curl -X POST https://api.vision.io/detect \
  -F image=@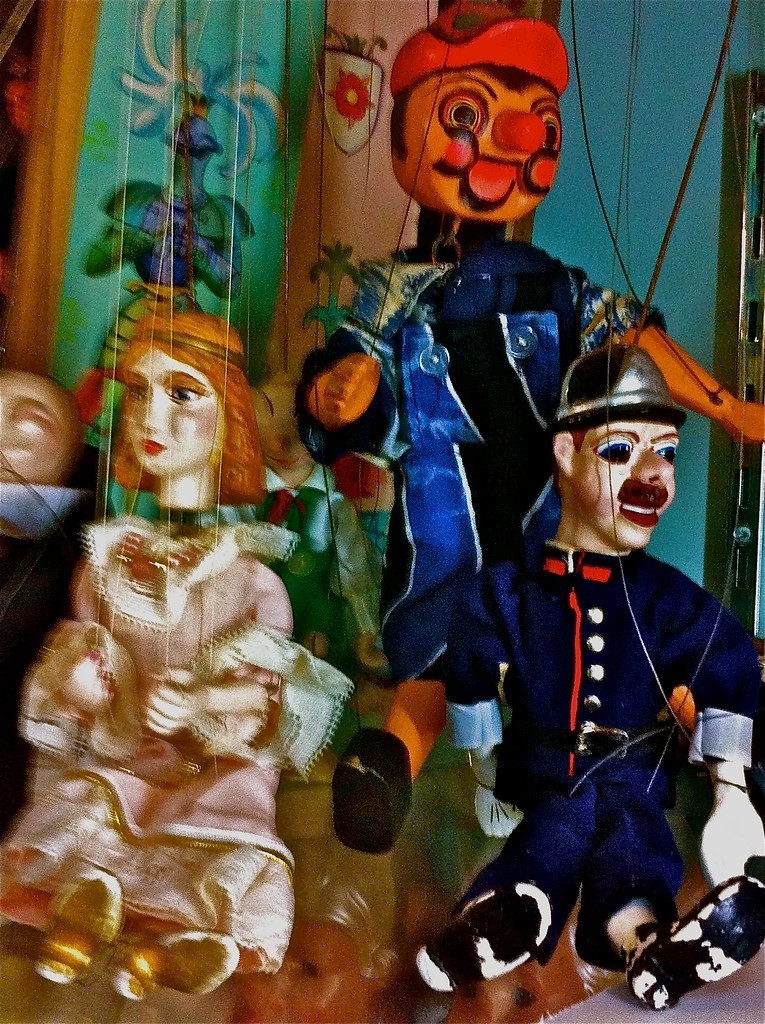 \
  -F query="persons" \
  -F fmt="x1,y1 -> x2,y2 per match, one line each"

417,339 -> 765,1011
0,312 -> 353,1001
293,2 -> 765,859
